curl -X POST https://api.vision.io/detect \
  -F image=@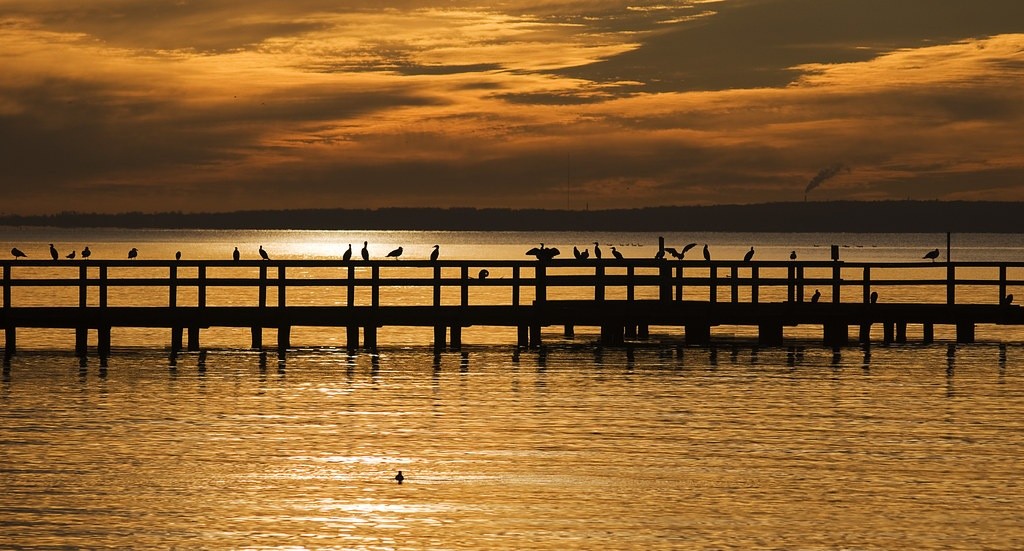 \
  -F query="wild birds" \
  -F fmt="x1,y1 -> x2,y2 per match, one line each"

430,245 -> 439,260
65,247 -> 91,259
573,242 -> 624,260
655,243 -> 711,261
233,247 -> 240,260
128,248 -> 138,259
790,251 -> 797,261
342,244 -> 352,260
871,291 -> 878,304
526,243 -> 560,260
176,251 -> 181,260
744,247 -> 754,261
49,244 -> 58,260
395,471 -> 404,480
259,245 -> 270,260
811,289 -> 821,303
11,248 -> 28,260
922,249 -> 939,262
385,247 -> 403,260
361,241 -> 369,260
